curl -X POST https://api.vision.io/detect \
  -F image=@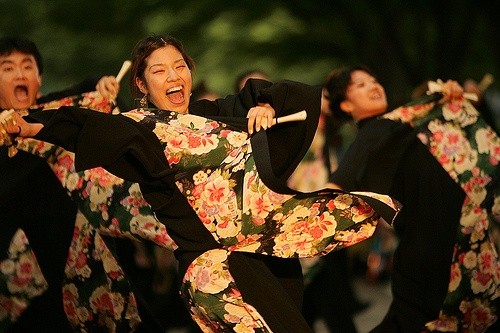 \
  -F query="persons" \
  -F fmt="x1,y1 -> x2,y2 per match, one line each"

0,33 -> 315,333
320,66 -> 500,333
235,64 -> 372,333
0,32 -> 124,333
190,69 -> 500,134
